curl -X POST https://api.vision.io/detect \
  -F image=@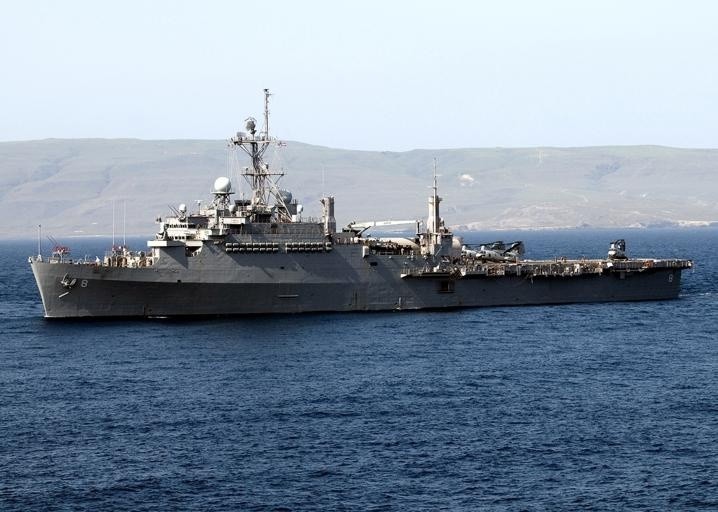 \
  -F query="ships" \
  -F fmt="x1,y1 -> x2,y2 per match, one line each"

28,84 -> 694,325
